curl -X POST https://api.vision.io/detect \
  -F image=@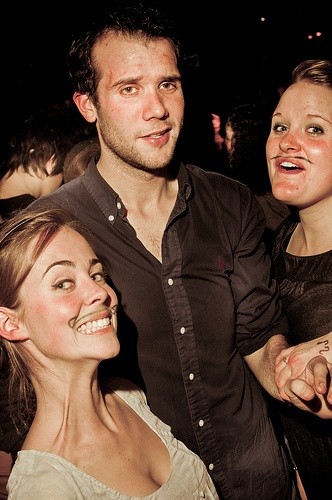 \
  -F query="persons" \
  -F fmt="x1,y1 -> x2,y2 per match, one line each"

7,12 -> 332,500
0,113 -> 294,241
258,55 -> 332,498
0,203 -> 217,500
